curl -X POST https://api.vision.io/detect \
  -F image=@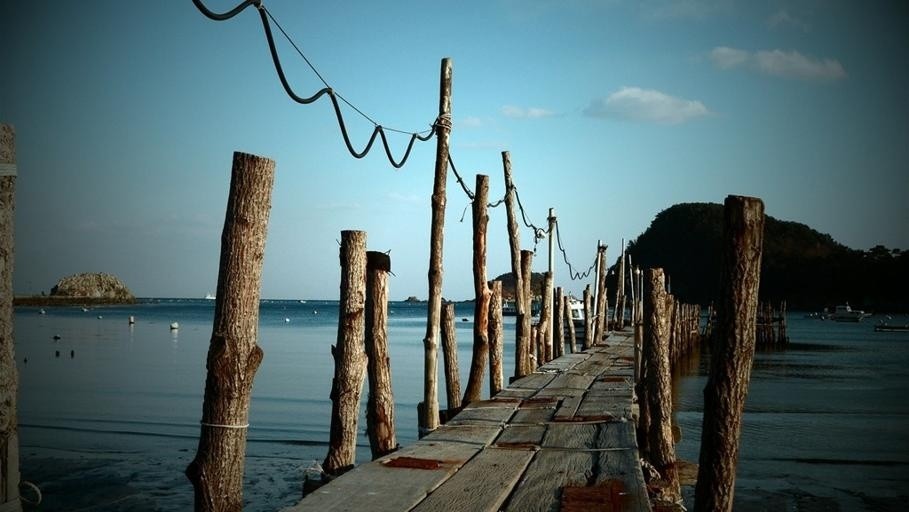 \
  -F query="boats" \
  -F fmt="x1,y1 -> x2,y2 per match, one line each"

496,296 -> 593,344
808,304 -> 909,335
